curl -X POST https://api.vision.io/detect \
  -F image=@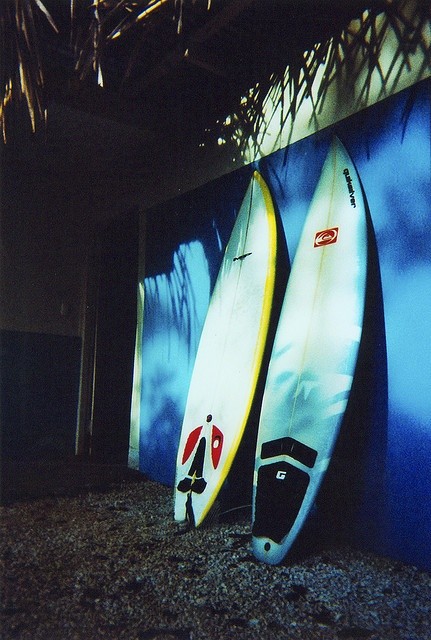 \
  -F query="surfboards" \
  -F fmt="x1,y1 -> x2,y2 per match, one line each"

249,136 -> 370,567
172,169 -> 282,533
